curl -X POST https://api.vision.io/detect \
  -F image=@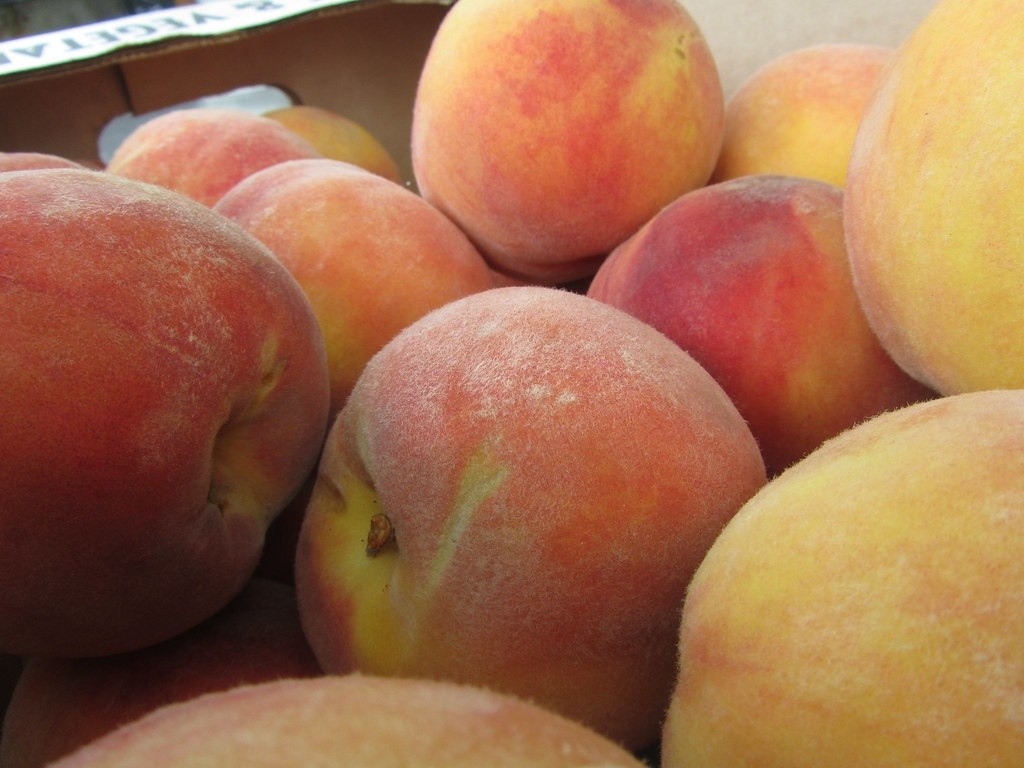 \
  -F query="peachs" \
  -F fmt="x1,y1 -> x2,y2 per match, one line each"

0,0 -> 1024,768
